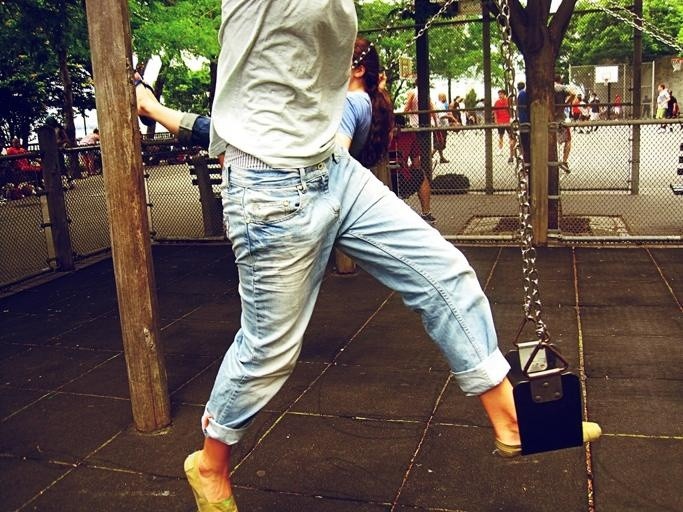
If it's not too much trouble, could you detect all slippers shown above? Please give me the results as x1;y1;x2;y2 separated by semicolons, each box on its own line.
134;68;158;126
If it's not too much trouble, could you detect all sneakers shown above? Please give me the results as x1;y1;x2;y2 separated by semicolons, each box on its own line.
561;162;570;173
439;157;450;164
494;421;602;458
184;450;237;511
421;212;437;224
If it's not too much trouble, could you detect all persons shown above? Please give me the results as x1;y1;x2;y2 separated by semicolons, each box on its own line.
1;116;101;199
132;36;393;171
181;0;602;511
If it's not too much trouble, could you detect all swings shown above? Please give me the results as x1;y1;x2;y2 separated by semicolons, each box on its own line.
496;0;583;455
